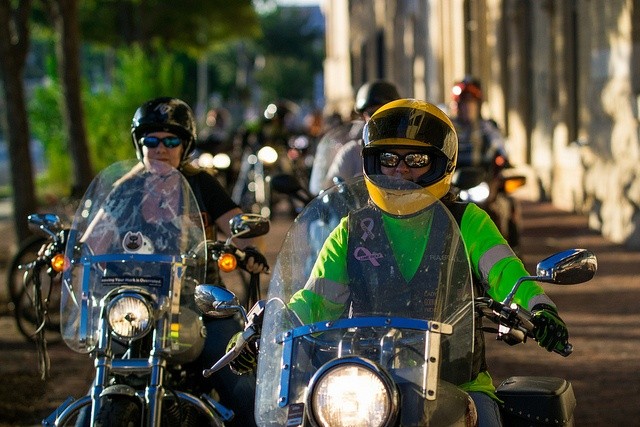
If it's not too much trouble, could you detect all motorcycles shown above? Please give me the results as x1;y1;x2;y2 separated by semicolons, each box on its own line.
263;169;367;282
193;171;599;427
16;157;273;427
447;153;527;250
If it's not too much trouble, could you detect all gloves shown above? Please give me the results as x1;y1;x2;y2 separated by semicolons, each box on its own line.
226;327;262;374
531;303;569;352
243;246;270;275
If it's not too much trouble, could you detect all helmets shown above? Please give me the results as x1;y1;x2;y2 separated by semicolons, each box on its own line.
355;80;400;116
362;98;459;218
131;97;198;157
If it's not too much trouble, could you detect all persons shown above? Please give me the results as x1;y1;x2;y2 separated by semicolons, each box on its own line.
40;96;271;427
224;98;570;426
441;76;519;249
309;81;404;196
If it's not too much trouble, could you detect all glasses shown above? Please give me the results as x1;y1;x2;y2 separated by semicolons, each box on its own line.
138;136;183;148
378;150;432;168
366;110;375;116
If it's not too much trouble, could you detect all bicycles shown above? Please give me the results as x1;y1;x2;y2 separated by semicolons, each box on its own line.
8;237;81;332
